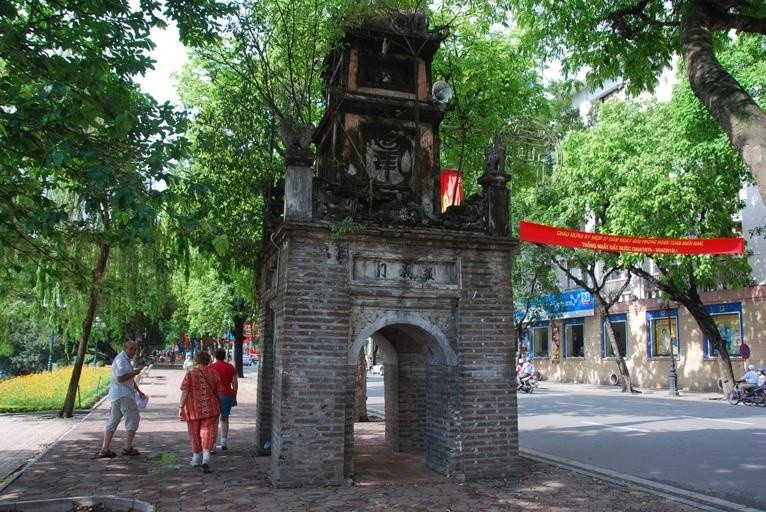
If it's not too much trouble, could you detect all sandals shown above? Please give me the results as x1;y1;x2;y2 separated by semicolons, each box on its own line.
98;448;118;458
120;446;140;456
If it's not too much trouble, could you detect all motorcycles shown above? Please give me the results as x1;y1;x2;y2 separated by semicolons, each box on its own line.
726;380;766;407
516;375;537;395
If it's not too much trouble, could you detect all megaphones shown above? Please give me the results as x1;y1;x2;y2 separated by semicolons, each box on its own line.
432;81;453;103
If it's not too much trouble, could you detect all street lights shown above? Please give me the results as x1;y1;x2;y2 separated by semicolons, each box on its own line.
655;297;682;398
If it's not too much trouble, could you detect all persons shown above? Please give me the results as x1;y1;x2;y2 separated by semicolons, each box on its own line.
98;340;145;458
516;358;534;390
739;364;766;400
178;349;237;473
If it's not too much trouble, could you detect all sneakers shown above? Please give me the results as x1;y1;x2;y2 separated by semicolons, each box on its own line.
191;457;212;474
